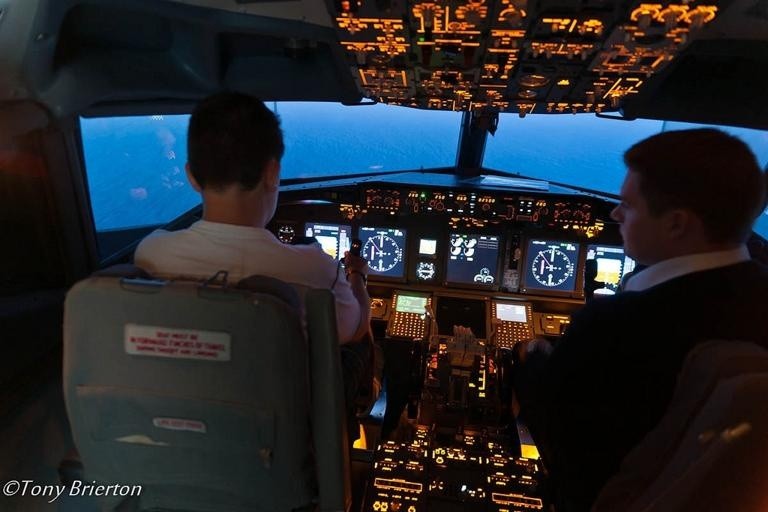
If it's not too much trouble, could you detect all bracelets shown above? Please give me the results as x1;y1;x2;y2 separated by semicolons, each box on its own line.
346;267;369;287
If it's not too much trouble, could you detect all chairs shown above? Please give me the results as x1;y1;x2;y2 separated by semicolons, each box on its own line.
587;340;767;509
58;275;357;511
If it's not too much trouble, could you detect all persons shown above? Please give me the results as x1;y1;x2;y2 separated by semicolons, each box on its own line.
511;124;767;510
134;88;387;417
